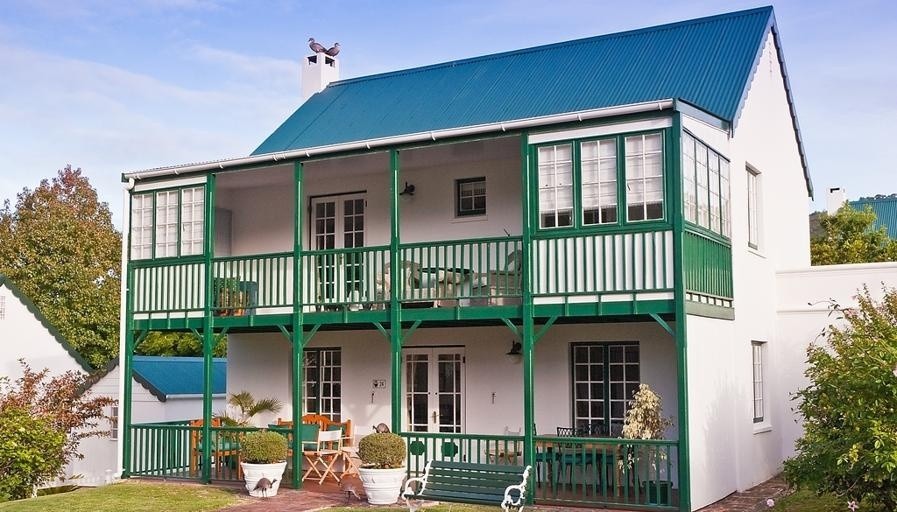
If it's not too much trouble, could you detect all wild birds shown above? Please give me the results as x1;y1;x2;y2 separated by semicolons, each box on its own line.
325;40;341;57
252;478;278;499
308;36;326;54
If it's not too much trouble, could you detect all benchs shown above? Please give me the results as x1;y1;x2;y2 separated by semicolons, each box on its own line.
188;417;244;479
400;458;532;511
275;414;351;478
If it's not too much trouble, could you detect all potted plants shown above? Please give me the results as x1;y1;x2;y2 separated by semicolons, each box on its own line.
237;430;288;497
355;432;407;504
616;383;674;505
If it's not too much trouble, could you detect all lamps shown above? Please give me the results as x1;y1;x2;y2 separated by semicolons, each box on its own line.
504;339;523;363
399;179;416;203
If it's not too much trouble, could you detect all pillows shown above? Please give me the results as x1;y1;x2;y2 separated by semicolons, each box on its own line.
267;423;319;450
194;428;241;451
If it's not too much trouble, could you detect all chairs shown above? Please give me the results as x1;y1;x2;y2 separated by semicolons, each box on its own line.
301;423;374;484
383;250;523;308
484;422;641;497
